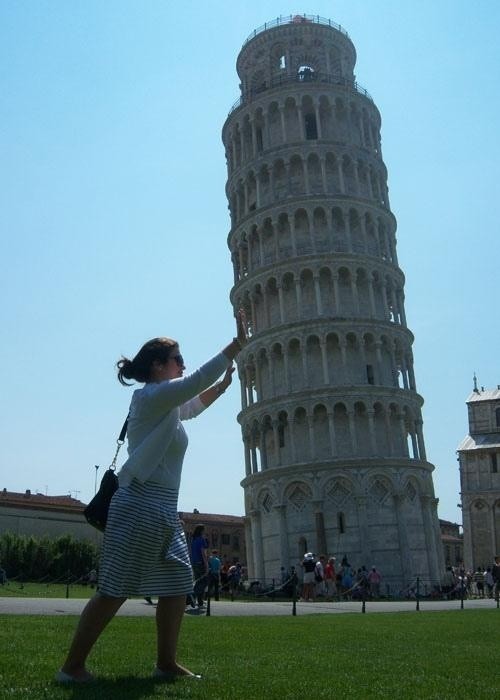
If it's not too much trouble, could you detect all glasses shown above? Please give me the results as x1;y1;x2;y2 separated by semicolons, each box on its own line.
168;354;184;367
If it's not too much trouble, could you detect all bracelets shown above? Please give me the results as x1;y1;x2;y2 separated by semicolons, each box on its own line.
233;337;244;351
214;383;226;394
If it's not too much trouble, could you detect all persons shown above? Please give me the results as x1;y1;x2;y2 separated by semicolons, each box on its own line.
54;307;249;684
203;548;500;602
144;595;153;605
187;523;209;609
87;568;97;588
79;567;90;586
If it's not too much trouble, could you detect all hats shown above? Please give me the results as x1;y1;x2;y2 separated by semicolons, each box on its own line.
304;552;314;561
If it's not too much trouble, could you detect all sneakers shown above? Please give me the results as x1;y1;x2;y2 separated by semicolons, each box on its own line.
188;594;196;608
150;662;202;680
199;605;207;609
55;666;97;688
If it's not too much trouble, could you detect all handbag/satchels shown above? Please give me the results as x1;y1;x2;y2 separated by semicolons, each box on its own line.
314;574;323;583
83;469;119;532
